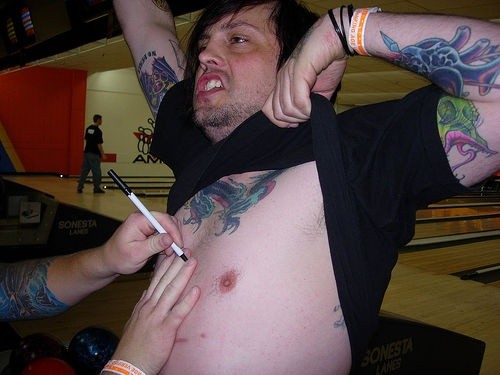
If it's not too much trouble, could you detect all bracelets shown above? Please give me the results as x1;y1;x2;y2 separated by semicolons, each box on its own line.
327;4;382;57
99;359;146;375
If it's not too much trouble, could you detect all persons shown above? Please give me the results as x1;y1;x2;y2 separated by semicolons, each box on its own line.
0;211;202;375
113;0;500;375
77;114;106;194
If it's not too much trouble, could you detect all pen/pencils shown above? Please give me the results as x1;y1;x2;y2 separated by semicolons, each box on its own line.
108;169;189;264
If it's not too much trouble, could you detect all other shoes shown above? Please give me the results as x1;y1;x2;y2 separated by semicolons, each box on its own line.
78;186;82;193
94;189;105;193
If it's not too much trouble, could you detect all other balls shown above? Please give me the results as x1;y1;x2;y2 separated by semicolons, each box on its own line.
22;357;76;375
68;325;120;375
9;332;68;375
